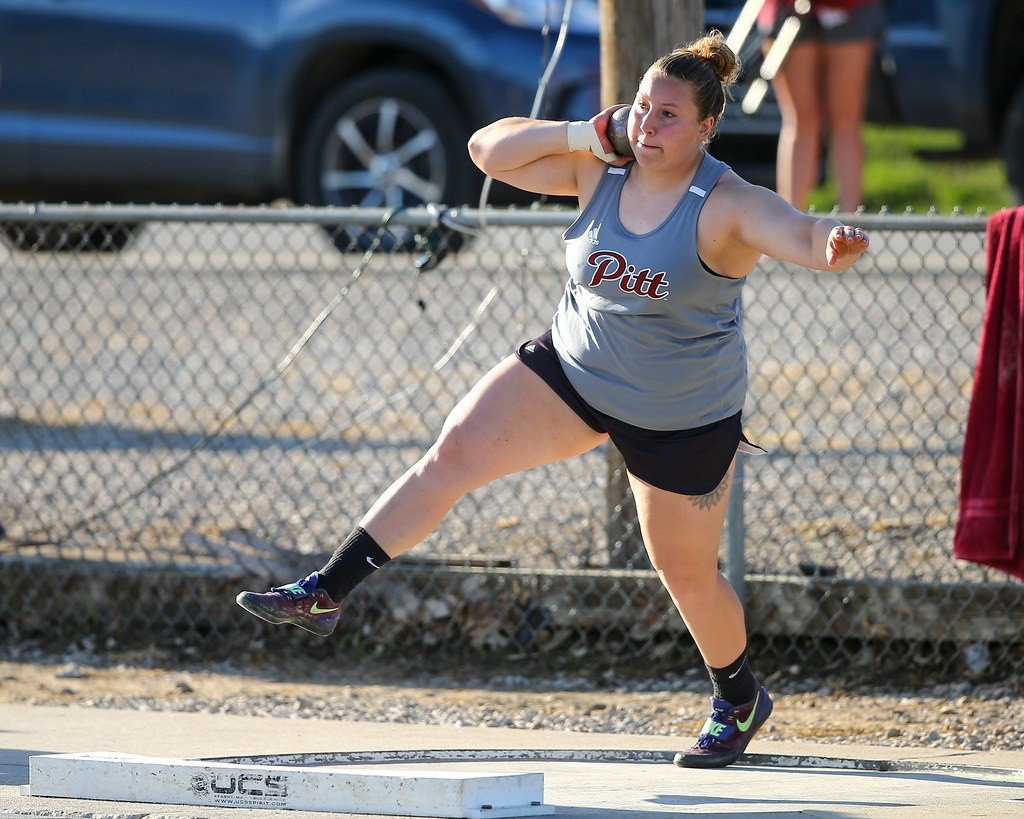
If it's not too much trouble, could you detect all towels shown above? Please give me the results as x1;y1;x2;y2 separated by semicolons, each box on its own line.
952;205;1024;582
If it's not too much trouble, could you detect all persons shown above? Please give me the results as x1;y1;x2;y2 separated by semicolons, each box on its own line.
756;0;882;212
237;30;871;772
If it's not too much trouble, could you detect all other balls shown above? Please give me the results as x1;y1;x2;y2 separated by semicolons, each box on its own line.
606;105;635;157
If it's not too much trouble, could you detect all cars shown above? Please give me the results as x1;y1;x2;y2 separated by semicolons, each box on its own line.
702;0;991;190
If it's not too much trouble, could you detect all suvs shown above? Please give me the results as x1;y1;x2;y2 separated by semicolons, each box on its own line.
0;0;600;254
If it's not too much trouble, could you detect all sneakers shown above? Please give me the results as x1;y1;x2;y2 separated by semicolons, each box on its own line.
672;679;773;768
236;571;343;638
